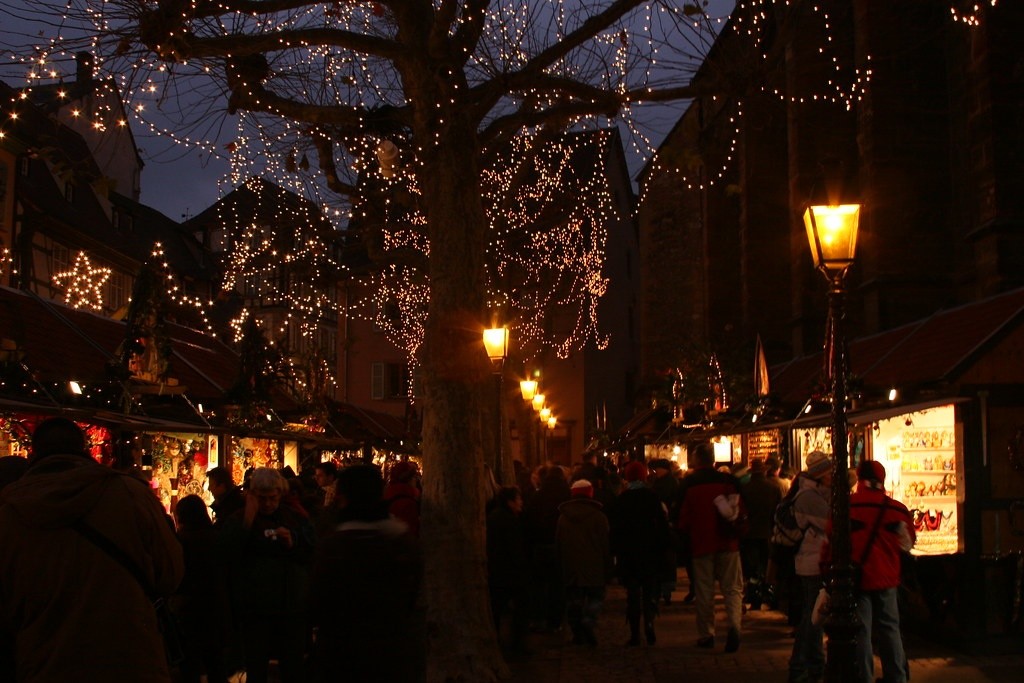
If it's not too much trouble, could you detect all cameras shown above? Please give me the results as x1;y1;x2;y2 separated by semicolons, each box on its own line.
264;529;278;541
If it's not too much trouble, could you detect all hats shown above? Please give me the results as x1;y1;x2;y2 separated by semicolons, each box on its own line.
856;457;886;489
807;452;835;479
746;454;783;478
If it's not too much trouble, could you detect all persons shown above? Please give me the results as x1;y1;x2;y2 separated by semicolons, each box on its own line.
0;418;915;683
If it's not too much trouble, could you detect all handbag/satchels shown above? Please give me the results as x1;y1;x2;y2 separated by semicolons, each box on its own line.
819;558;871;599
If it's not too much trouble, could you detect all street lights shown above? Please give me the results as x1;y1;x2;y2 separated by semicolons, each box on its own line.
520;379;558;468
483;327;511;491
803;193;868;683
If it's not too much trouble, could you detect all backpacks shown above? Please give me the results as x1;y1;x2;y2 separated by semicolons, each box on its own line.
768;484;833;571
712;491;756;542
